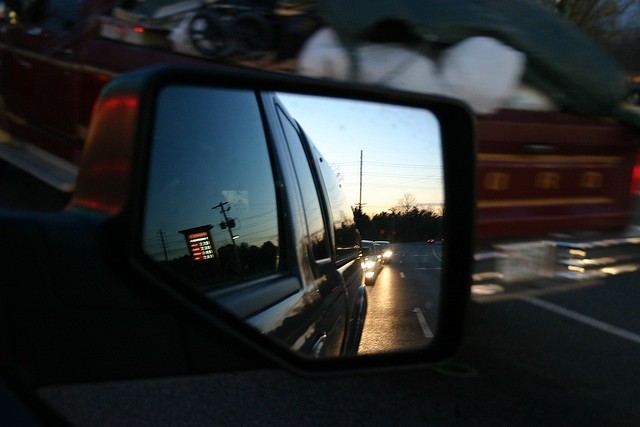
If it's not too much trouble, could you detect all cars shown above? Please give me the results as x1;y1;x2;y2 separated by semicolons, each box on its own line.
0;0;639;306
373;242;383;267
143;87;367;354
357;239;377;284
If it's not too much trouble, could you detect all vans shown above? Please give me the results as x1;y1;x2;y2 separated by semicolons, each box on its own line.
375;239;392;263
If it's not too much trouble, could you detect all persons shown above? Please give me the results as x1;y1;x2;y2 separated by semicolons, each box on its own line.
227;15;323;73
